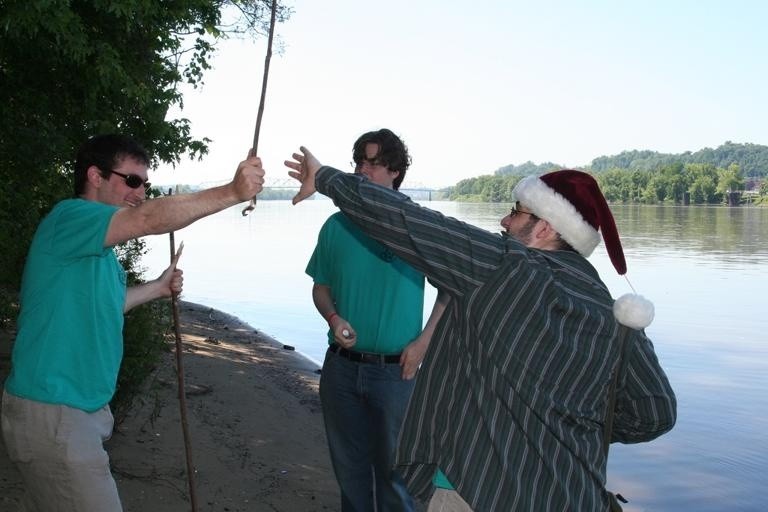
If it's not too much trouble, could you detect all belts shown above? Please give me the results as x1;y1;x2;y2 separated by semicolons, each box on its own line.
328;342;401;365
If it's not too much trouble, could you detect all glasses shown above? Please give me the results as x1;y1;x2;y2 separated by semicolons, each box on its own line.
100;166;152;194
349;159;388;171
510;207;540;220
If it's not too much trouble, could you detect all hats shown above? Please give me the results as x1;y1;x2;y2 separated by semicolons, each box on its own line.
509;168;657;333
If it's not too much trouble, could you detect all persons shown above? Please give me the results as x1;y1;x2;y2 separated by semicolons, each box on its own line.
0;134;266;512
284;146;677;512
305;130;452;512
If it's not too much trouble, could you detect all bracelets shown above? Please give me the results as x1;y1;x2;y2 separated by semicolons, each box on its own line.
327;313;338;326
